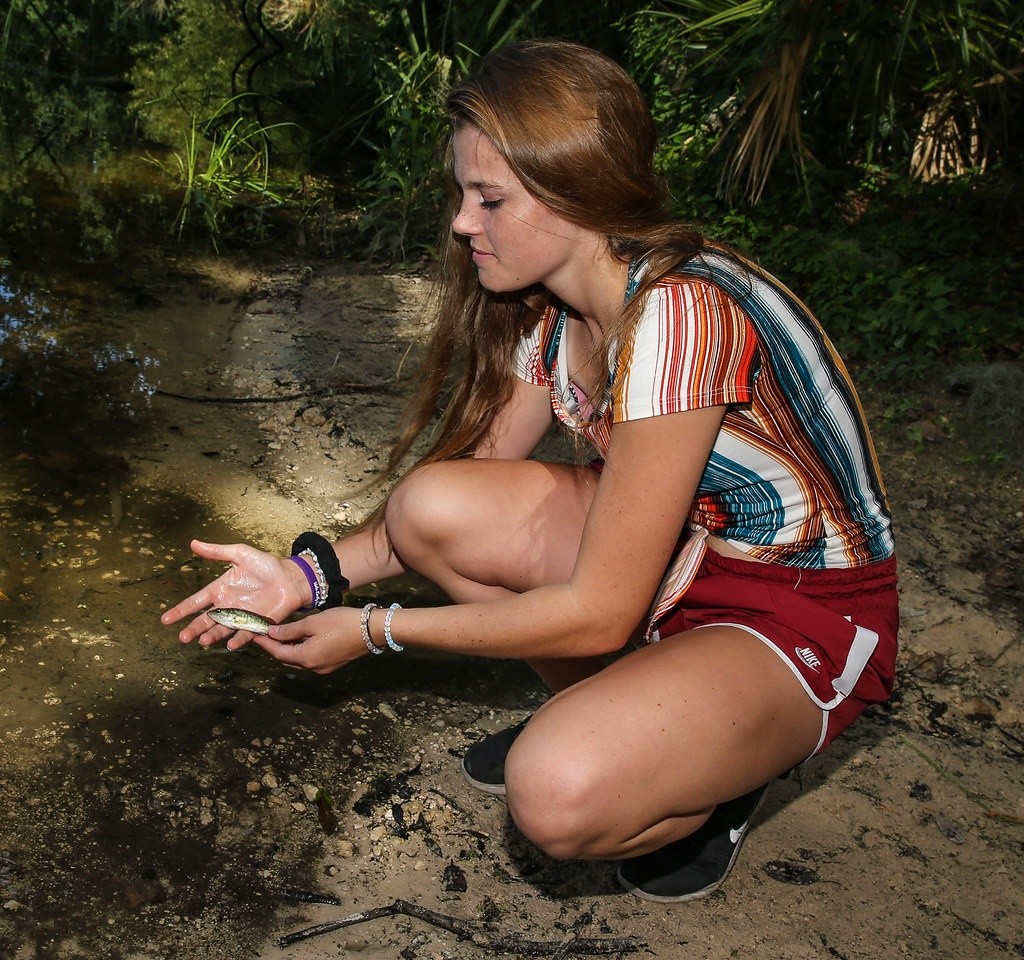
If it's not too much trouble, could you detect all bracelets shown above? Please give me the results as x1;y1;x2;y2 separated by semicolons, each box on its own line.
298;548;329;606
384;603;404;652
360;603;385;654
287;556;321;614
291;531;350;610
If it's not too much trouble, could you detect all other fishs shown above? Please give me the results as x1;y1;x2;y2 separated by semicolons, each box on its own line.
208;608;278;635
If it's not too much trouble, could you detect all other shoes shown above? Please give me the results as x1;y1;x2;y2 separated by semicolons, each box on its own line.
464;705;539;791
616;779;772;904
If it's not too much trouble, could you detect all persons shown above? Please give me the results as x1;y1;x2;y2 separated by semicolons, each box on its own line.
160;35;901;904
